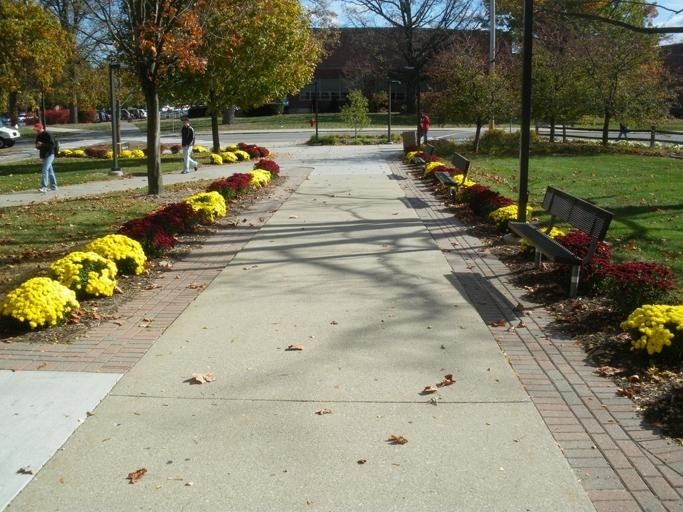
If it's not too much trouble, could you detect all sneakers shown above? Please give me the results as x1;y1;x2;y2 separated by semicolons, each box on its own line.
36;185;57;193
180;161;198;174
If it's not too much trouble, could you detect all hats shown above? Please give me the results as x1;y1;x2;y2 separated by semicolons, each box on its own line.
31;122;44;132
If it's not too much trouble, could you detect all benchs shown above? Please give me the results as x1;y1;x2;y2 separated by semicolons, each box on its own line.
507;186;615;297
434;152;469;203
412;143;433;165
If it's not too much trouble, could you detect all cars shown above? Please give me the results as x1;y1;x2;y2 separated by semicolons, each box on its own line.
0;111;34;149
96;105;190;123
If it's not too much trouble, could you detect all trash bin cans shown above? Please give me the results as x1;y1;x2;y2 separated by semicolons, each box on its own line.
400;131;418;152
222;109;235;124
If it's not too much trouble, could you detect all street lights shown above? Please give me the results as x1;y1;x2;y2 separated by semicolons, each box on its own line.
388;78;401;142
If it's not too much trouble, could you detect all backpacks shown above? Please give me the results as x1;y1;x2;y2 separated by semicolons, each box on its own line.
52;135;60;157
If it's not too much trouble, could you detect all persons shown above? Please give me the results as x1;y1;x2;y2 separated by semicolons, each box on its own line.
615;123;628;142
180;116;198;174
420;111;431;144
32;122;57;193
